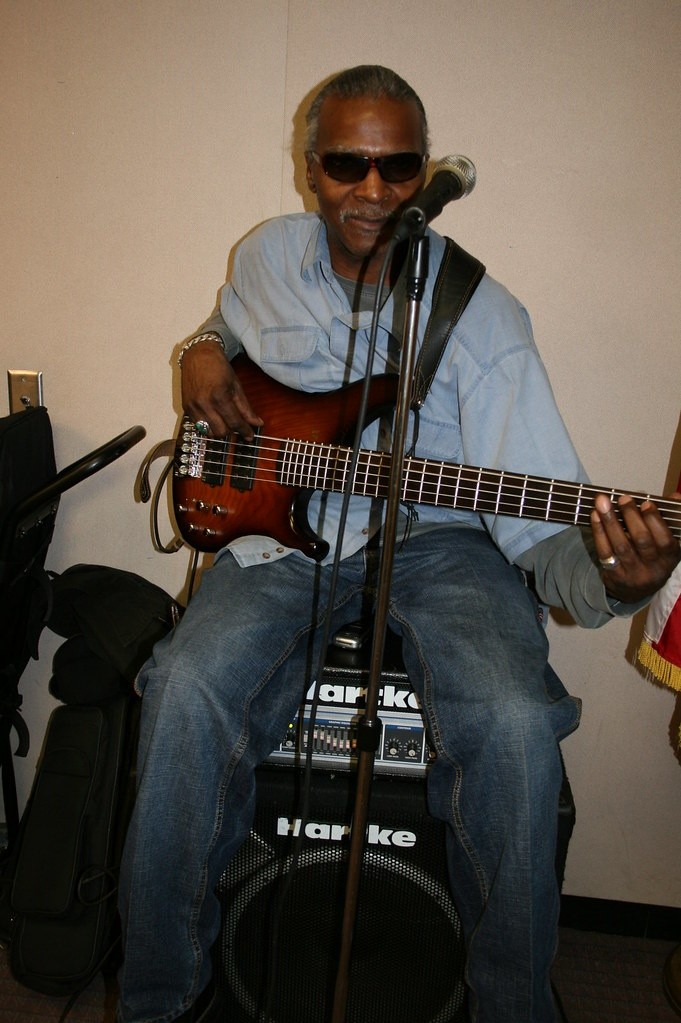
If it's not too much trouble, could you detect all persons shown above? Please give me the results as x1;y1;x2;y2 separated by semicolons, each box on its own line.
112;66;681;1023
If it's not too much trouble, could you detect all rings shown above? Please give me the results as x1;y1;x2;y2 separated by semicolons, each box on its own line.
196;420;209;433
600;554;618;569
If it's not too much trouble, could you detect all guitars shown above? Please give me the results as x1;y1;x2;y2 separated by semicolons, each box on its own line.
168;345;680;565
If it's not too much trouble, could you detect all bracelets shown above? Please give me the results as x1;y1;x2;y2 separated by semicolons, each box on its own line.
177;334;224;368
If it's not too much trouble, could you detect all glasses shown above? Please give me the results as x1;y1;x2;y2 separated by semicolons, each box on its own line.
312;150;426;184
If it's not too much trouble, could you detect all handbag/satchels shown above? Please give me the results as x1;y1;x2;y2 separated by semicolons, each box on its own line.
44;562;184;678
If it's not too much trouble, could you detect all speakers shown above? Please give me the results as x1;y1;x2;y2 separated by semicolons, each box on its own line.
195;760;577;1023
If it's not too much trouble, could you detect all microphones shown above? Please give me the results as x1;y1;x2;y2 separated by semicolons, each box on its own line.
393;155;477;244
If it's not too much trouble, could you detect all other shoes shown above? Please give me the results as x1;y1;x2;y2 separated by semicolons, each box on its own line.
172;978;223;1023
